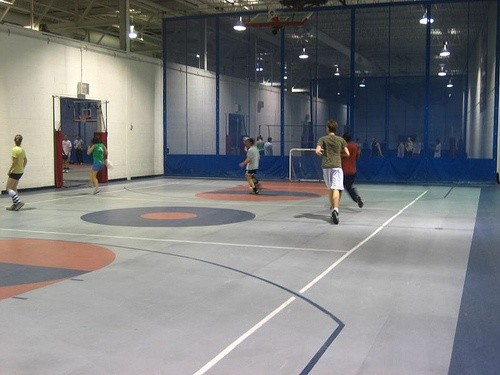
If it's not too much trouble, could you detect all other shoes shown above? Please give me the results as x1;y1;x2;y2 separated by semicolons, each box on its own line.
356;196;363;207
63;169;65;173
66;170;69;173
94;188;101;194
6;204;17;210
15;201;24;211
332;210;339;224
254;189;259;194
254;182;259;190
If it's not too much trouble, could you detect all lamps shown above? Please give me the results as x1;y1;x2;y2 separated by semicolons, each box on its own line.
299;48;308;59
419;9;433;24
234;16;246;31
440;42;450;57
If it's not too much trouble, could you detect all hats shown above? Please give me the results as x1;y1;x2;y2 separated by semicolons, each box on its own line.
76;136;80;139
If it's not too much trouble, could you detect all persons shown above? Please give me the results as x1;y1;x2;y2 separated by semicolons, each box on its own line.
355;136;443;160
87;136;107;194
73;135;85;164
5;134;27;211
239;137;260;194
264;137;273;156
315;120;350;224
256;136;265;155
61;134;72;173
339;133;364;208
